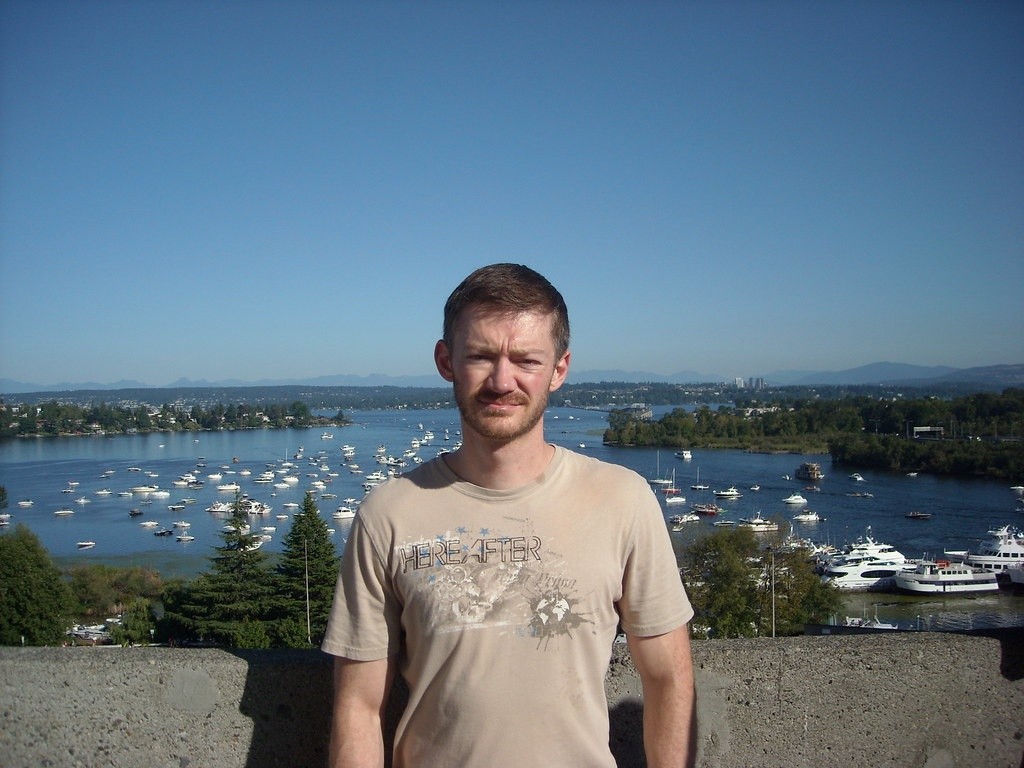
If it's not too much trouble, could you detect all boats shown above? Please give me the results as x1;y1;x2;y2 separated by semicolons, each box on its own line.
68;481;79;487
1009;485;1024;514
0;514;12;526
906;472;918;477
895;552;1001;593
99;474;109;478
92;488;114;496
646;446;908;596
53;510;75;516
17;499;34;506
904;511;931;520
942;525;1024;587
104;467;116;474
74;498;91;504
119;425;464;554
77;546;93;551
61;488;74;493
76;542;95;546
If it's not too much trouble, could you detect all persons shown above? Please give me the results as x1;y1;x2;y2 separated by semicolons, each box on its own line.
320;263;696;768
